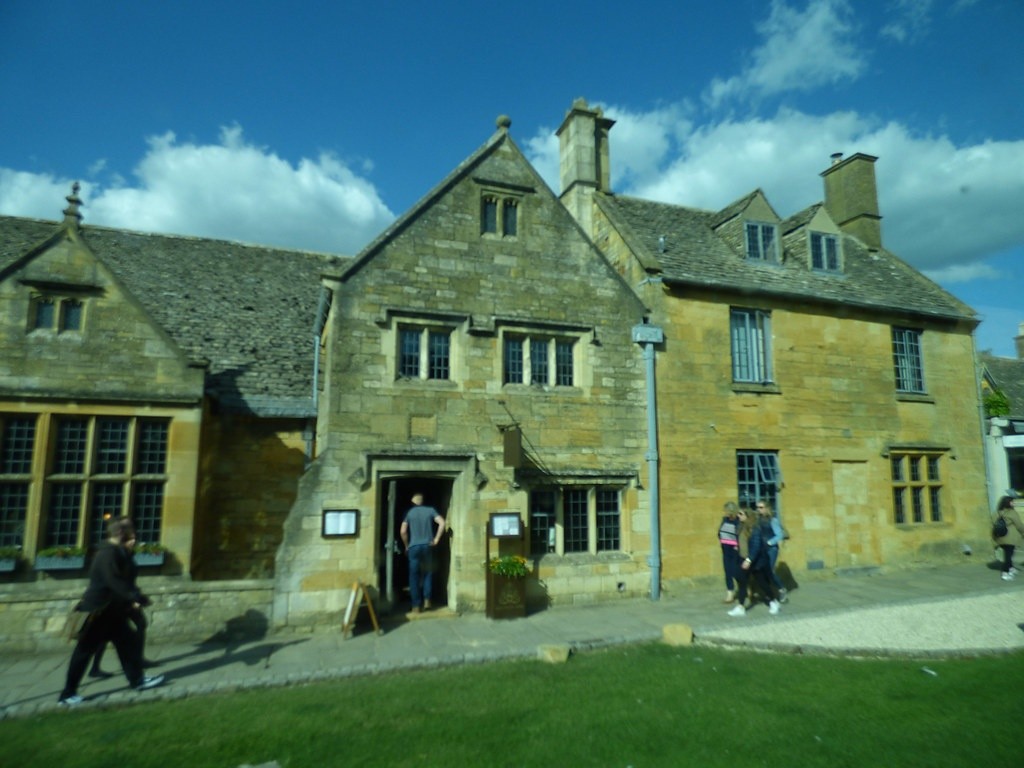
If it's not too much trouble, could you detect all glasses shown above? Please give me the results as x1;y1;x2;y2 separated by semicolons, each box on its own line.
739;513;746;515
756;506;764;509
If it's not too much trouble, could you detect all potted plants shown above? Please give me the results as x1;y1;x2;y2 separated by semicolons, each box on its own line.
483;554;534;595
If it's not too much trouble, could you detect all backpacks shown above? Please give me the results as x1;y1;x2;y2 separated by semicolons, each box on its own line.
993;510;1013;536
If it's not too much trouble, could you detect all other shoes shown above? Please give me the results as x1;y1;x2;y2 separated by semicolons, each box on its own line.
142;660;156;667
89;669;113;678
58;696;82;706
411;606;421;613
424;600;431;608
1009;567;1019;574
743;597;751;608
136;675;164;690
728;606;745;616
768;600;780;614
1001;571;1012;580
726;590;737;603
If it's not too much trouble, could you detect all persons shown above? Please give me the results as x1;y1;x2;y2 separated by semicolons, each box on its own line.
755;500;787;594
728;507;779;617
401;493;445;614
55;516;165;707
717;502;756;603
990;497;1024;581
